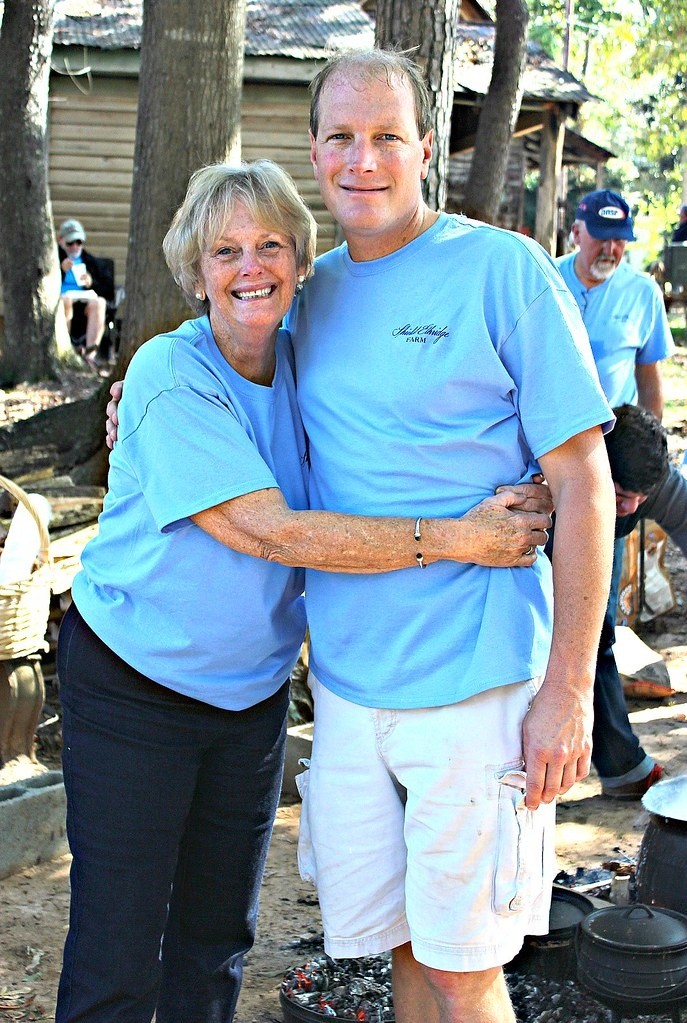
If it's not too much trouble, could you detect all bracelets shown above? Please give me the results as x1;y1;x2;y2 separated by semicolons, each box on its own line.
413;517;427;569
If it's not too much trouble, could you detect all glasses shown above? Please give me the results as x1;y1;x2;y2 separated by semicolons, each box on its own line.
66;240;82;245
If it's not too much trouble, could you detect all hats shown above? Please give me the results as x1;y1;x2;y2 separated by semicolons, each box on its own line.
576;187;635;241
60;219;85;244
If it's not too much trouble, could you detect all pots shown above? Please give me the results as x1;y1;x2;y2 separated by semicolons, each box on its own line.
631;774;686;917
576;905;687;1014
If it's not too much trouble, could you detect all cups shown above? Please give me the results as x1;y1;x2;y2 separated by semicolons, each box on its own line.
71;263;87;286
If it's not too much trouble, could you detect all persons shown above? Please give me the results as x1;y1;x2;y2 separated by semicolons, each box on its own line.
557;189;687;796
26;159;553;1022
57;219;106;369
104;48;616;1023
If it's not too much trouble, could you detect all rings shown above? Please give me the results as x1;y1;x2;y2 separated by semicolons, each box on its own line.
525;545;534;555
539;528;549;546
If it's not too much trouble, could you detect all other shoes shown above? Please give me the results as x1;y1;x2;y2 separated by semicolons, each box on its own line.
603;762;672;798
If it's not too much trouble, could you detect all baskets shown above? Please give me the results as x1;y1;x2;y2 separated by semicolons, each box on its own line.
0;474;53;661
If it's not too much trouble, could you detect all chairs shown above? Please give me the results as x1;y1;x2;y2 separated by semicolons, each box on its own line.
70;257;118;362
